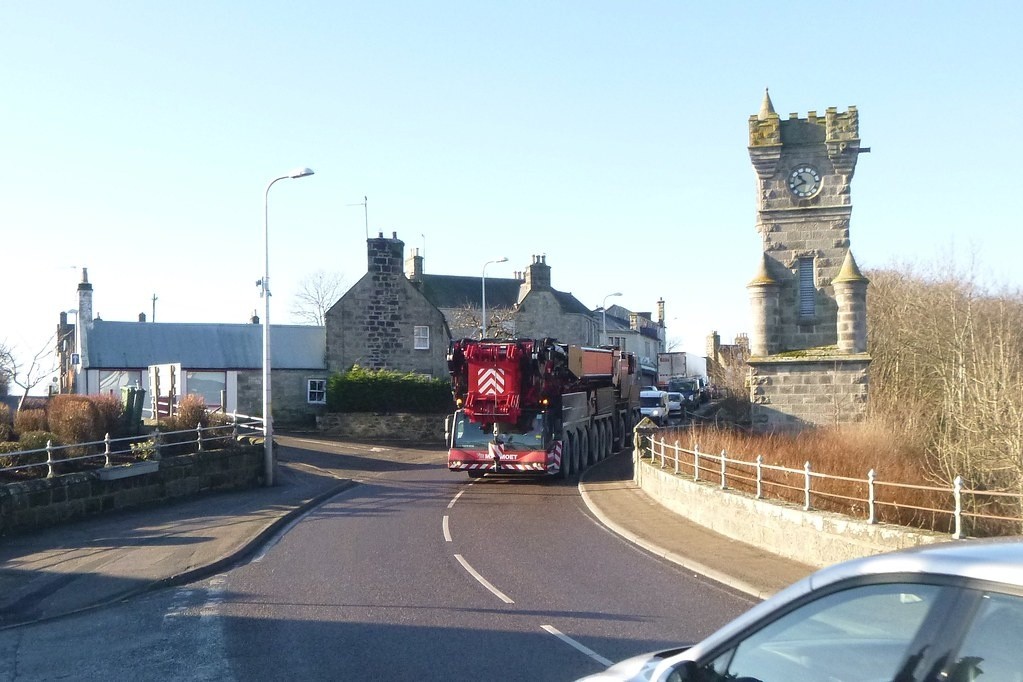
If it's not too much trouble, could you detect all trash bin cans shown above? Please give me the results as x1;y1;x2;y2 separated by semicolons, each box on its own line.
120;385;146;425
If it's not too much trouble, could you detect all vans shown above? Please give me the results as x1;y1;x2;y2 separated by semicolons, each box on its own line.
638;390;671;427
641;386;658;392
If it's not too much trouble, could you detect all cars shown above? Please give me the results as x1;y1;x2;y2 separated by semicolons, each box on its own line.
667;391;686;412
567;531;1023;682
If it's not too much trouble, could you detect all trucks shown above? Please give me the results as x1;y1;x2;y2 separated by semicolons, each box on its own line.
669;377;712;412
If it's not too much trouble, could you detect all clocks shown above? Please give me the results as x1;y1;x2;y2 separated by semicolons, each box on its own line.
788;166;820;197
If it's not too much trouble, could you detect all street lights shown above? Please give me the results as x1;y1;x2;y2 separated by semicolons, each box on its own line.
481;258;508;338
603;293;623;344
261;168;315;487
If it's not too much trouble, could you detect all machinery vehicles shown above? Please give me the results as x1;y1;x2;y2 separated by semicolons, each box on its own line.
443;335;641;480
